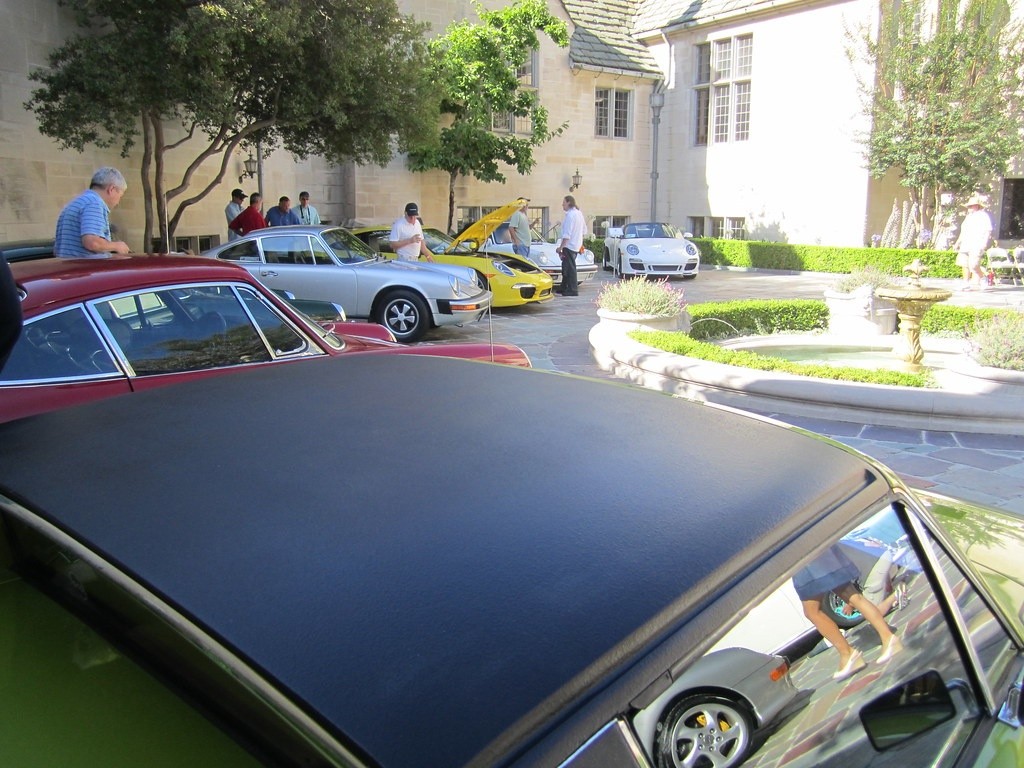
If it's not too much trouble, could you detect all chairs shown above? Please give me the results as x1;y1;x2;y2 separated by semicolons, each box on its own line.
267;247;279;263
293;243;305;264
985;246;1024;286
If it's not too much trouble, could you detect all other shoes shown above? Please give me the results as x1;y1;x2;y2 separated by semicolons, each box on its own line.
562;291;577;296
556;288;565;293
894;581;908;609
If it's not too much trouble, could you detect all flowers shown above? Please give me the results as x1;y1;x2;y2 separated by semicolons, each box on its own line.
591;271;688;317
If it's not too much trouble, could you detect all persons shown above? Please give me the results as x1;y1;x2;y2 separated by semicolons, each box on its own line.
292;191;321;225
264;196;304;226
54;167;131;257
556;195;587;296
389;203;435;263
954;196;992;291
225;189;248;242
230;192;268;237
0;252;24;379
509;196;541;256
792;540;908;680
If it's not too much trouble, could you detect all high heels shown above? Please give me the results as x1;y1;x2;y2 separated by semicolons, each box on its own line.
833;649;865;683
876;634;904;664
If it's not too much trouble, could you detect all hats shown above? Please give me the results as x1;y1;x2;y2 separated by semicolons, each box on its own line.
518;197;530;201
405;203;418;215
232;189;248;199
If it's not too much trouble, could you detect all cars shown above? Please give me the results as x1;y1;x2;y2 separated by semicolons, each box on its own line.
0;240;346;348
697;527;913;658
602;222;700;279
198;225;494;344
305;197;554;307
0;353;1023;768
632;647;797;768
477;222;598;288
1;258;533;424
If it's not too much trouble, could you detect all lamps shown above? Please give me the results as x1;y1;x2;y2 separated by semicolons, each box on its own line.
569;168;582;192
238;150;256;183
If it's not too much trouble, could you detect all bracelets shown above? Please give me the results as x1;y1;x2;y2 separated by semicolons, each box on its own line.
426;255;430;258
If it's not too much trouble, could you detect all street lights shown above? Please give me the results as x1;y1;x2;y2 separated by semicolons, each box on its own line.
650;93;664;222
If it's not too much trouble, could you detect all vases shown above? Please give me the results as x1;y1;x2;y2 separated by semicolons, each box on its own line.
595;307;677;331
